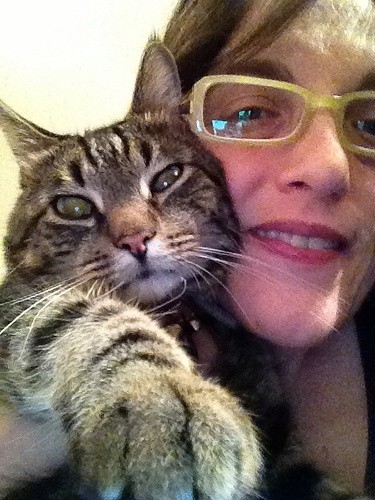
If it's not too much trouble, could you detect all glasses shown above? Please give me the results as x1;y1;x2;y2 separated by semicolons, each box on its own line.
179;75;375;158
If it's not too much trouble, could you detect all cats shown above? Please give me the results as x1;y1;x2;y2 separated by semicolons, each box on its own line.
0;27;354;500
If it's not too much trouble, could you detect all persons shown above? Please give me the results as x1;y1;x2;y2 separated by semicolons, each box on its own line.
0;0;375;500
